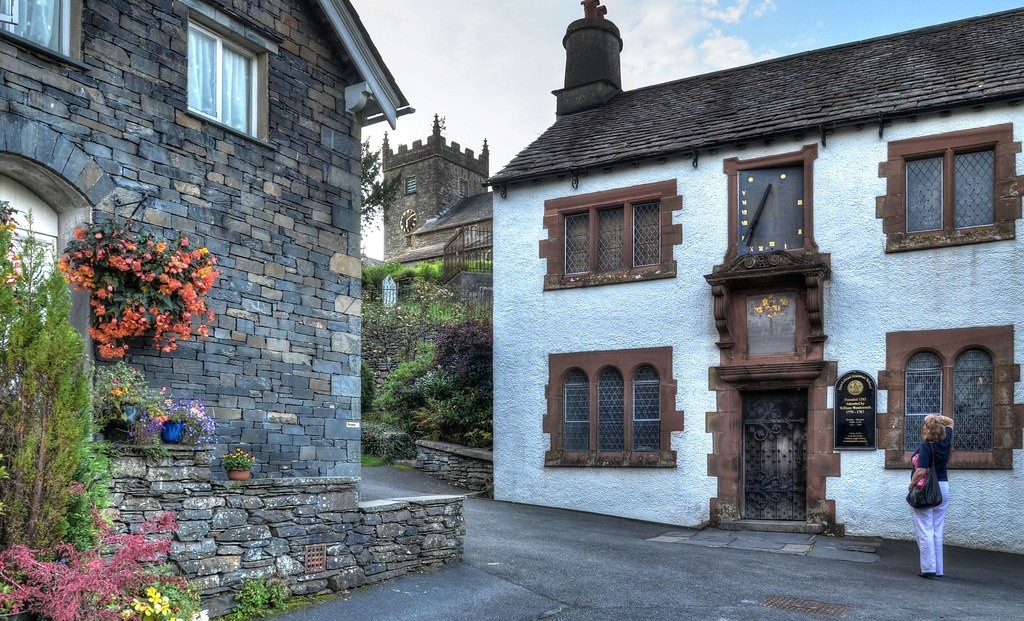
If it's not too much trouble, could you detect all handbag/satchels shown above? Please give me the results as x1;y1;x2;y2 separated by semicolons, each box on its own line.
905;442;943;509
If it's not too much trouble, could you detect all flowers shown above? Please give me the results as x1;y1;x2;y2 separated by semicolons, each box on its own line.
117;391;222;465
220;447;256;470
86;360;165;431
58;217;221;360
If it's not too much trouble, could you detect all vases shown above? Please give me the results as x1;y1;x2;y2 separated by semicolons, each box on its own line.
228;469;250;480
161;421;185;444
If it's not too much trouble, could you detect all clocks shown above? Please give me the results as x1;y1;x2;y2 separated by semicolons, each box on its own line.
400;209;417;232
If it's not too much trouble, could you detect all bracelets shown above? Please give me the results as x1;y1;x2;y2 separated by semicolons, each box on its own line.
941;416;944;423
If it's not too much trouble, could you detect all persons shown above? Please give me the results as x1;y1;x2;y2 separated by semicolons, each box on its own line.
905;414;955;580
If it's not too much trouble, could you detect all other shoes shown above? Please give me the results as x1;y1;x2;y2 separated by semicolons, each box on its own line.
918;572;936;578
